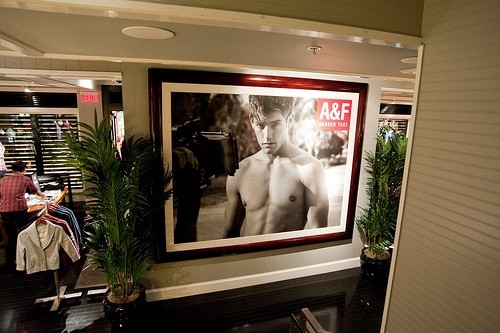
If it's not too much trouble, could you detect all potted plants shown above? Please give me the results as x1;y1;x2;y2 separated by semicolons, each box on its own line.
356;124;409;283
54;107;174;333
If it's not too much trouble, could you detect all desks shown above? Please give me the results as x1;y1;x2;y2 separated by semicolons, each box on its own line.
24;188;68;212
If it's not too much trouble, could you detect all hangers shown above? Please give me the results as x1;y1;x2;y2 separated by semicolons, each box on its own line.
33;202;59;226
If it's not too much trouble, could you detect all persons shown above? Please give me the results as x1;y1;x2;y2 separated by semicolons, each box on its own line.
0;142;46;261
222;95;330;238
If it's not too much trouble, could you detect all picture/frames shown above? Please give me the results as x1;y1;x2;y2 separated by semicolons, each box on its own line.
148;67;369;264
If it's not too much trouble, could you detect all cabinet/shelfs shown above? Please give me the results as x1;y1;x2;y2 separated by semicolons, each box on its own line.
378;119;408;139
